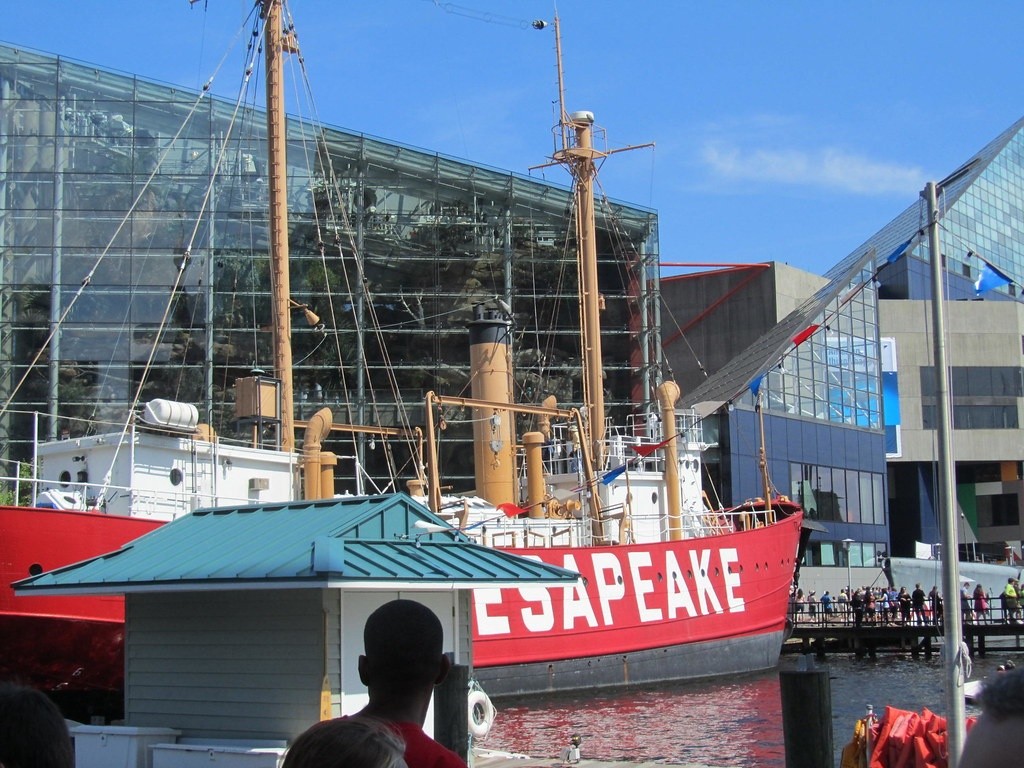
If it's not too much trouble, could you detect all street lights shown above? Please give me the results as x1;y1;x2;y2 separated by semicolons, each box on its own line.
843;538;856;601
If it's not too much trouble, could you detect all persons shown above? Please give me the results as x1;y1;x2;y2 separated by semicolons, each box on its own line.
280;599;469;767
795;577;1023;626
1;668;76;768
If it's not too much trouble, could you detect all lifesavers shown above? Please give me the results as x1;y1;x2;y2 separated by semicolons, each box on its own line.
466;690;495;737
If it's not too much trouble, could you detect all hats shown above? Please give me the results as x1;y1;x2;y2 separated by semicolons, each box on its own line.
810;591;816;596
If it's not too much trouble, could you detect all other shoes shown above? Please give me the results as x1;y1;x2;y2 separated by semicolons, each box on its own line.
809;618;815;622
814;618;818;622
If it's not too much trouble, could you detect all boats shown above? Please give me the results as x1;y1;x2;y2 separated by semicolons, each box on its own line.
1;0;804;700
